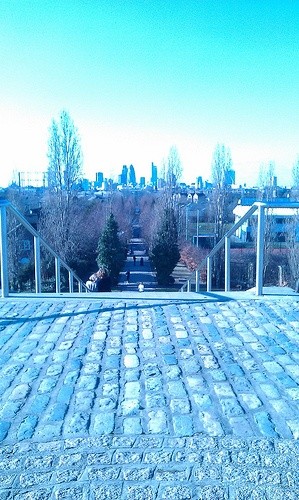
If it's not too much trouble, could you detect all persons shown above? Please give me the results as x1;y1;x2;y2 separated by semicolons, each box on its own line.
137;282;145;292
133;256;137;265
125;270;130;281
140;257;144;265
